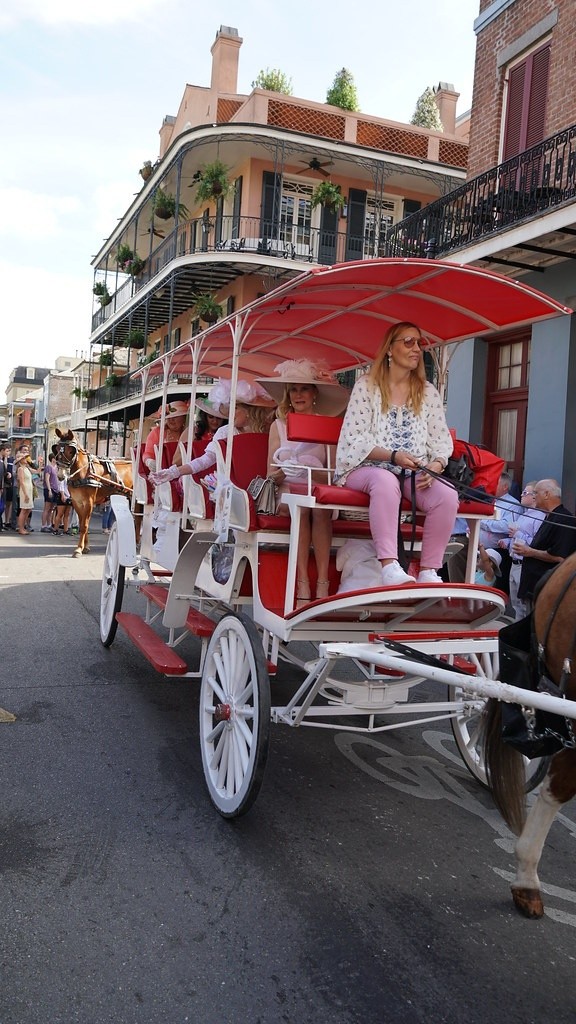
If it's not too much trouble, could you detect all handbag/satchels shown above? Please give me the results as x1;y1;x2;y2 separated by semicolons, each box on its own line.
246;475;277;516
442;457;473;493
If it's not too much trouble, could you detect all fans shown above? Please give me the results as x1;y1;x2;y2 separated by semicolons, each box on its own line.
295;157;336;178
138;227;165;239
180;170;203;188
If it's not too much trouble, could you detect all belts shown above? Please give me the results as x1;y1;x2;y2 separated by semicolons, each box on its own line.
512;560;523;564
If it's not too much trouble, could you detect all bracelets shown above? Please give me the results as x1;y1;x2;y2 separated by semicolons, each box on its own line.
391;450;398;466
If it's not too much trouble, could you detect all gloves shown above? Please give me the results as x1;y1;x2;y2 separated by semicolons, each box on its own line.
147;464;179;485
145;459;158;471
281;460;301;478
289;466;306;479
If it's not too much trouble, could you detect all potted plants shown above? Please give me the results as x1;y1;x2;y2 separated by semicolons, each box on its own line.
96;295;113;306
123;329;153;351
116;243;143;274
191;293;224;325
308;181;344;211
195;160;238;207
136;160;154;178
104;373;122;387
92;282;109;295
152;189;192;223
98;354;117;366
72;386;95;398
136;351;158;367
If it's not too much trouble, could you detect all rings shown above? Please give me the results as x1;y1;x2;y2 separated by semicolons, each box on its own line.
428;483;431;487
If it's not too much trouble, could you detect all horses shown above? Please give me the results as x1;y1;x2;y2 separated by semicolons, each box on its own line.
54;428;144;558
483;552;576;920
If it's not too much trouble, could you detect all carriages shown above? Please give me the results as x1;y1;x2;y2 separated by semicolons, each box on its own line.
98;256;576;920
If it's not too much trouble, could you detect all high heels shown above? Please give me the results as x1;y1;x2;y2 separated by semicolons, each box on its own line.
296;580;311;609
315;580;329;600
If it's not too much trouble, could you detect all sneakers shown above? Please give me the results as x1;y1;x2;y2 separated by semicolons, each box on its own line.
417;569;444;584
381;561;416;586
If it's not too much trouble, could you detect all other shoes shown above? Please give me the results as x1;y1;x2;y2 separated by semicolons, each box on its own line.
0;519;114;535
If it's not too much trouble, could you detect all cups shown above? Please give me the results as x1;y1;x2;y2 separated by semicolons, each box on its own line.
514;538;526;545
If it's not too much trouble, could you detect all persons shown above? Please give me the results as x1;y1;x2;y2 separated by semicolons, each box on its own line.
42;453;80;536
148;377;278;502
171;397;229;493
438;472;523;596
253;358;351;609
512;479;576;601
497;481;547;620
0;443;44;535
142;401;189;473
102;507;115;534
333;320;459;585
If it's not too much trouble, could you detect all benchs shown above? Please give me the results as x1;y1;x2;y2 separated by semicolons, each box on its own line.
153;441;188;514
279;409;505;525
214;430;429;550
129;446;157;515
179;440;226;534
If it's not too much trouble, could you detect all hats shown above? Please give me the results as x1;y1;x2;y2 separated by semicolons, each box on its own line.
12;452;30;465
152;405;173;422
477;548;502;577
165;400;190;419
194;392;229;420
253;357;349;417
208;377;278;408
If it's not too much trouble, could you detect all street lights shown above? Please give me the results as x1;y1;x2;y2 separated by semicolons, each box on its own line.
42;417;49;479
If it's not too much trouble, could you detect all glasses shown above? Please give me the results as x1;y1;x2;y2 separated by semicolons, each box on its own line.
522;491;535;496
391;337;430;351
